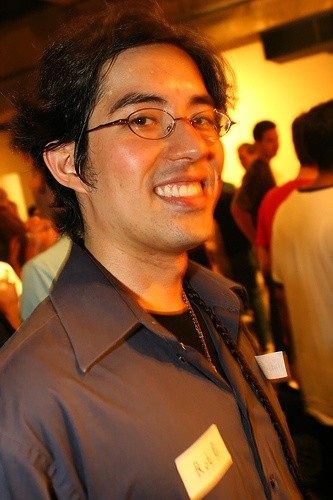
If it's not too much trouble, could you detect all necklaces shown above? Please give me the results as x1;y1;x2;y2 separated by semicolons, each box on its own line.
181;290;218;374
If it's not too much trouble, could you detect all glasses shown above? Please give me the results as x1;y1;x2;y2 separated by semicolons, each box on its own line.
88;107;236;141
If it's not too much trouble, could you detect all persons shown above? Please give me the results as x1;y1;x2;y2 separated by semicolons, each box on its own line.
189;99;333;500
0;184;73;348
0;10;312;500
